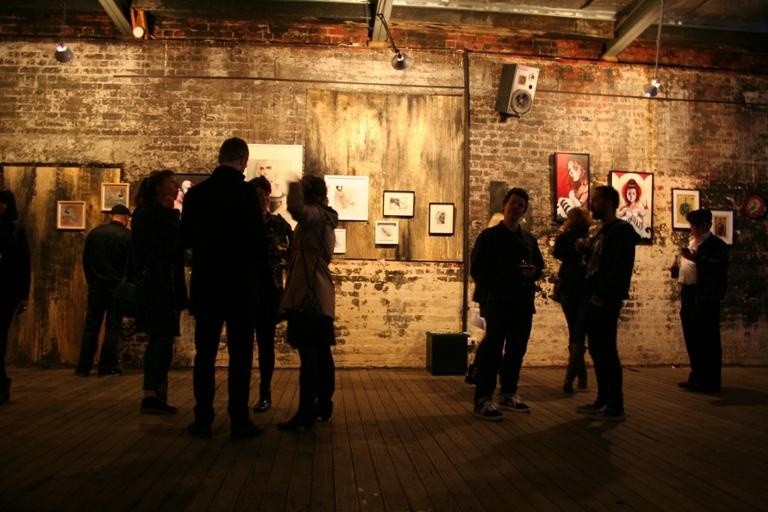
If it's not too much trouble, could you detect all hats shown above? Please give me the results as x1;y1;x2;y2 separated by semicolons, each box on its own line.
110;204;133;218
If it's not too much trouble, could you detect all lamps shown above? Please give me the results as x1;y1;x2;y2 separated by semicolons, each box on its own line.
376;13;412;70
644;1;664;98
57;2;73;63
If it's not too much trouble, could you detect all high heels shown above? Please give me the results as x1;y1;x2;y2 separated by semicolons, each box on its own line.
284;408;318;432
314;398;334;422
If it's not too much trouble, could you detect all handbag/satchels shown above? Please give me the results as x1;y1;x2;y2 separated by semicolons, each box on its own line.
111;276;149;318
286;302;328;350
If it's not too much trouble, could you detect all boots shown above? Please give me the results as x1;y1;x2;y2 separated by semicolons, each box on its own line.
252;382;274;414
0;377;14;405
563;343;587;393
577;362;587;389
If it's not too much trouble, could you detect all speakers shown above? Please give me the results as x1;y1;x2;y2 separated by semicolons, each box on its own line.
495;64;540;117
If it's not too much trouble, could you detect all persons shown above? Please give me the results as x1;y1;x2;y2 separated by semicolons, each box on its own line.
669;206;729;394
463;212;505;389
0;163;32;403
616;178;648;238
556;155;588;220
573;184;641;422
74;138;337;442
547;206;593;396
469;186;544;422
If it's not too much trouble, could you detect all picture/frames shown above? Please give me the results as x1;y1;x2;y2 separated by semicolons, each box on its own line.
332;228;346;255
243;143;304;232
554;152;590;222
171;174;211;221
709;207;734;245
382;190;415;218
608;170;654;245
56;201;86;231
323;175;369;221
375;221;399;245
671;188;700;231
429;203;455;235
101;182;130;212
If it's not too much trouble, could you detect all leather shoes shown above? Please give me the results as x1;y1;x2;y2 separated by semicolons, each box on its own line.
230;423;264;441
186;419;213;439
141;396;179;416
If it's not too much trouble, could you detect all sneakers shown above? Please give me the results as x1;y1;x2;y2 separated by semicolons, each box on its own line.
74;367;90;378
584;405;625;420
677;380;721;394
498;393;529;412
97;367;122;377
575;404;601;414
474;396;504;420
